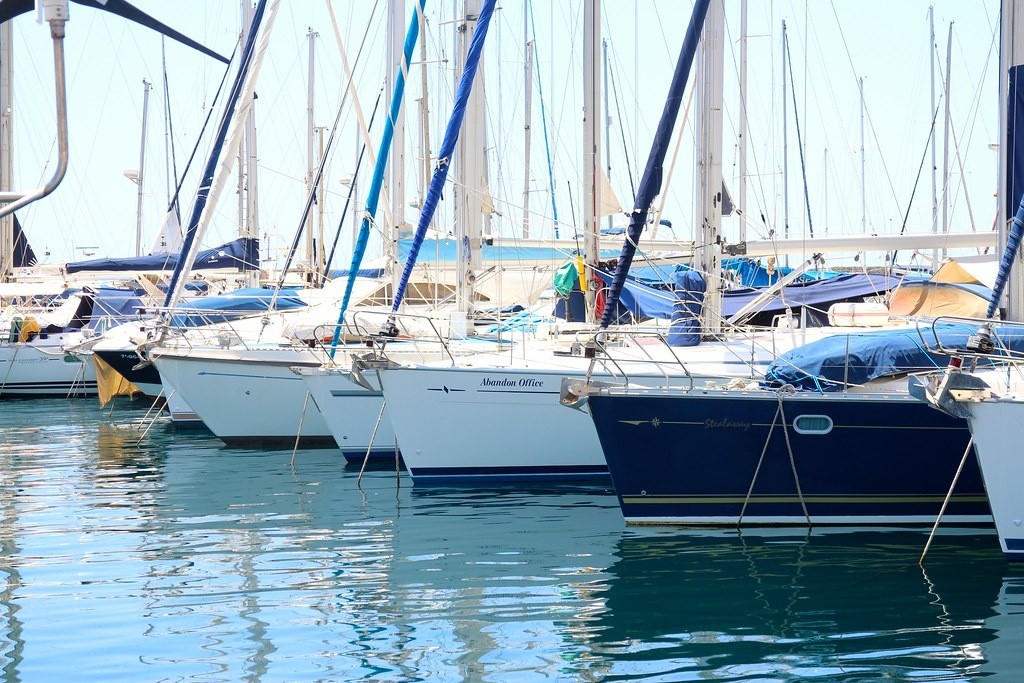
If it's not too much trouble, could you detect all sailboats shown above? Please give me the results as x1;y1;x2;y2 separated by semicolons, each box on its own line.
0;0;1024;557
